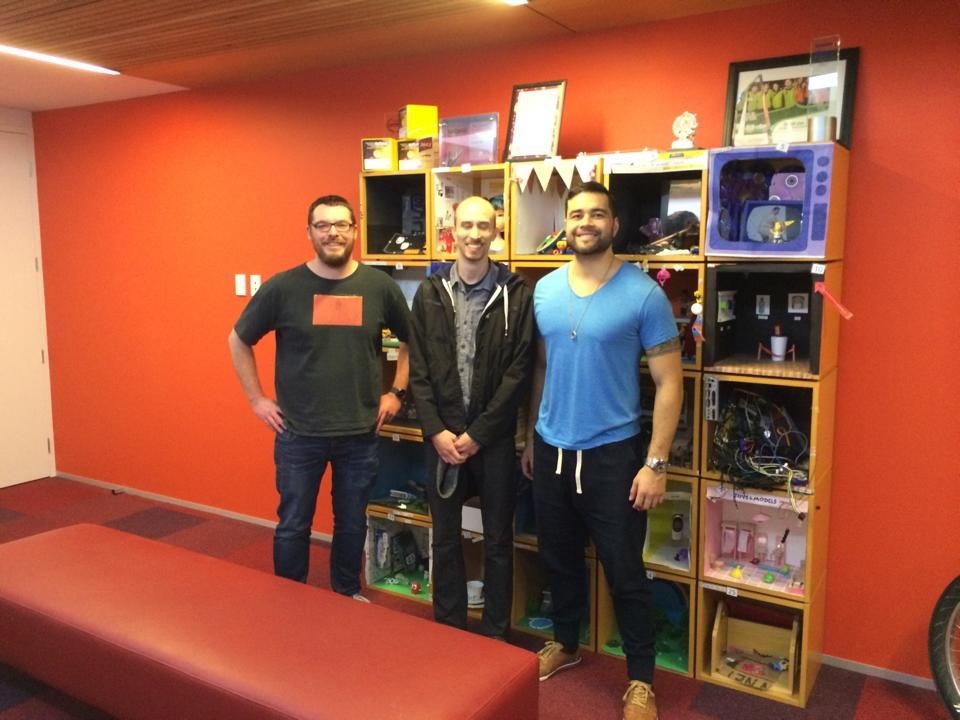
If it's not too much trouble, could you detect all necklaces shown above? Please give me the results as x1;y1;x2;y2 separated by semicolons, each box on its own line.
568;253;615;339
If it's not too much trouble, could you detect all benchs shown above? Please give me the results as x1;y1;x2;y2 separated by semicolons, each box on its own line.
1;521;539;720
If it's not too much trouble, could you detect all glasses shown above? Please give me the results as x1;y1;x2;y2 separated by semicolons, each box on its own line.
310;221;352;232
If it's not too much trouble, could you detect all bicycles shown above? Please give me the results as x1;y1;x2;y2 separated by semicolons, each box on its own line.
929;574;960;720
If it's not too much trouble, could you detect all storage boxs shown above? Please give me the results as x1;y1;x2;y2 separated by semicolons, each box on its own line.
361;137;399;171
398;139;440;170
399;104;438;139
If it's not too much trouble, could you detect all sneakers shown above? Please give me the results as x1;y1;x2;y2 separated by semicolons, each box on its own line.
622;680;659;720
535;639;582;681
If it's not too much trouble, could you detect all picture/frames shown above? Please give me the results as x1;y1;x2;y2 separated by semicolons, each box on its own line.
723;47;862;148
439;112;499;166
502;78;567;162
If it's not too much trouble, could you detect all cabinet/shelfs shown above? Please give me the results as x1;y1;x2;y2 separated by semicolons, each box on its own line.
358;141;852;709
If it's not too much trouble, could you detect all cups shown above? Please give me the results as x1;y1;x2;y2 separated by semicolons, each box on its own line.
466;581;484;603
771;336;788;361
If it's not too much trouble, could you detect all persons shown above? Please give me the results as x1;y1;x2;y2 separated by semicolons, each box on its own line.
228;195;409;603
521;181;682;720
410;196;536;642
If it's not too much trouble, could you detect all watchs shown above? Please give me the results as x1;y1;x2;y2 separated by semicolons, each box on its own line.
644;457;670;474
388;386;408;405
